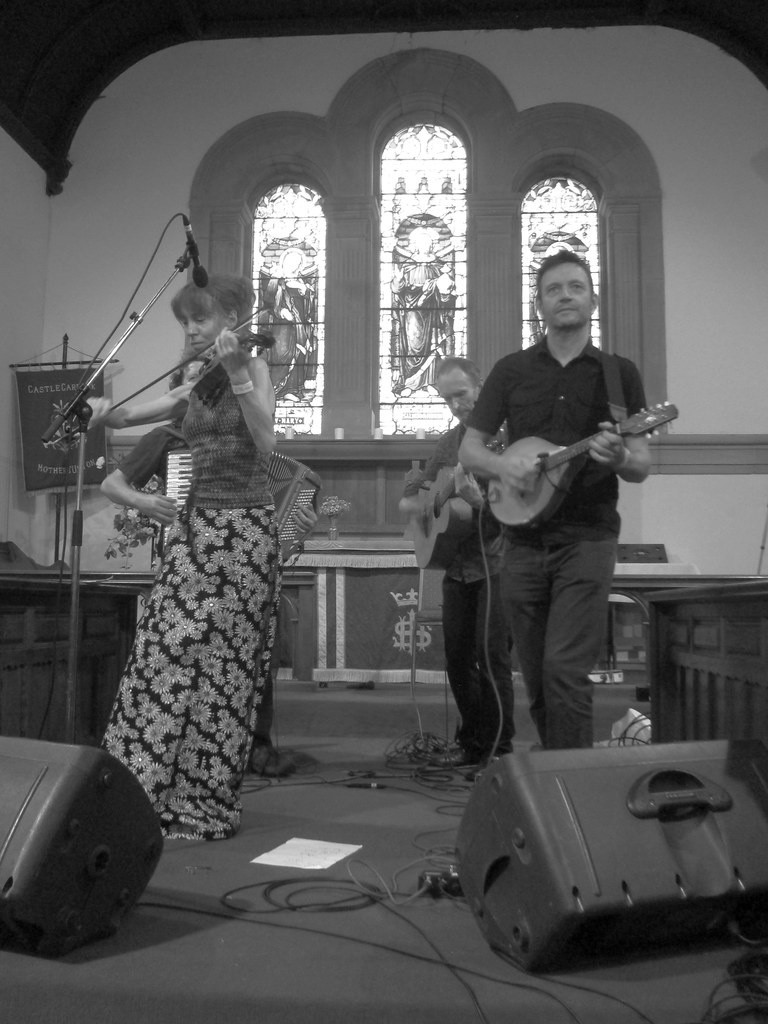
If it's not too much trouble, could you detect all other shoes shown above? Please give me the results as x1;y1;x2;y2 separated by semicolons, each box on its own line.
431;749;473;767
247;746;296;777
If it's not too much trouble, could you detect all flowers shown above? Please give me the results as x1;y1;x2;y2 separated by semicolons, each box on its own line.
318;494;350;516
94;455;165;571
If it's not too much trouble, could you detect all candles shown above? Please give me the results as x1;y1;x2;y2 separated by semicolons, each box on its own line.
374;428;383;440
334;426;345;440
285;428;296;441
415;429;425;440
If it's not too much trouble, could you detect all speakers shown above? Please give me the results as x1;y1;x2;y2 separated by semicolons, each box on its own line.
0;736;163;960
453;737;768;977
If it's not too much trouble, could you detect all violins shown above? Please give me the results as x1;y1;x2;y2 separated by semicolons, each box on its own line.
192;330;277;398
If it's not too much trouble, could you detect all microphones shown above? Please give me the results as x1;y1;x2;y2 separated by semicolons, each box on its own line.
181;215;209;288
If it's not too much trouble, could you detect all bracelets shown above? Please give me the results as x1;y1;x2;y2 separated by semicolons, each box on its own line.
613;446;631;473
232;380;254;394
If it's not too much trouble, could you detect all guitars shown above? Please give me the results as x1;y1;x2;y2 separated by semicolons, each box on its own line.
409;437;506;572
486;400;678;535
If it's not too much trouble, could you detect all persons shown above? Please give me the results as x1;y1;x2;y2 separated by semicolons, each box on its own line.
399;357;509;780
458;252;653;749
81;273;317;840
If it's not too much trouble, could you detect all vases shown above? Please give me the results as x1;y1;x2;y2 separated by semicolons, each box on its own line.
324;513;343;540
